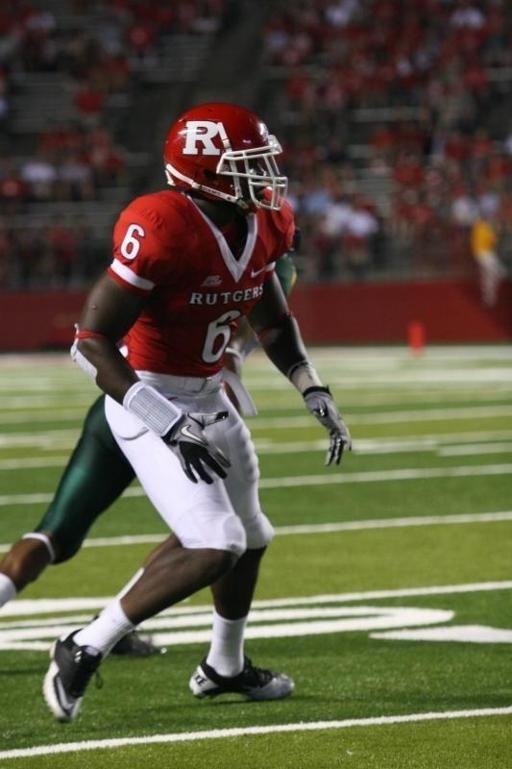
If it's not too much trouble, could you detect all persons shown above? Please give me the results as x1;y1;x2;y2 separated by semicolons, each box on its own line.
41;101;355;723
0;251;300;656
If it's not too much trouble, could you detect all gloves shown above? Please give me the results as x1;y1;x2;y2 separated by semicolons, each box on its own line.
160;411;233;484
302;387;352;467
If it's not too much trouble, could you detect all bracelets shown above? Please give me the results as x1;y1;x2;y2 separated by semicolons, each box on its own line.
121;380;182;438
286;359;324;395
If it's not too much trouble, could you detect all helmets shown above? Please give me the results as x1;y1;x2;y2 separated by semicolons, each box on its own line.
162;102;290;211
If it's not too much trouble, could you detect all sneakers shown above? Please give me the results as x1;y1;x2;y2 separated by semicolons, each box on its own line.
41;627;103;722
90;615;168;657
188;655;295;701
